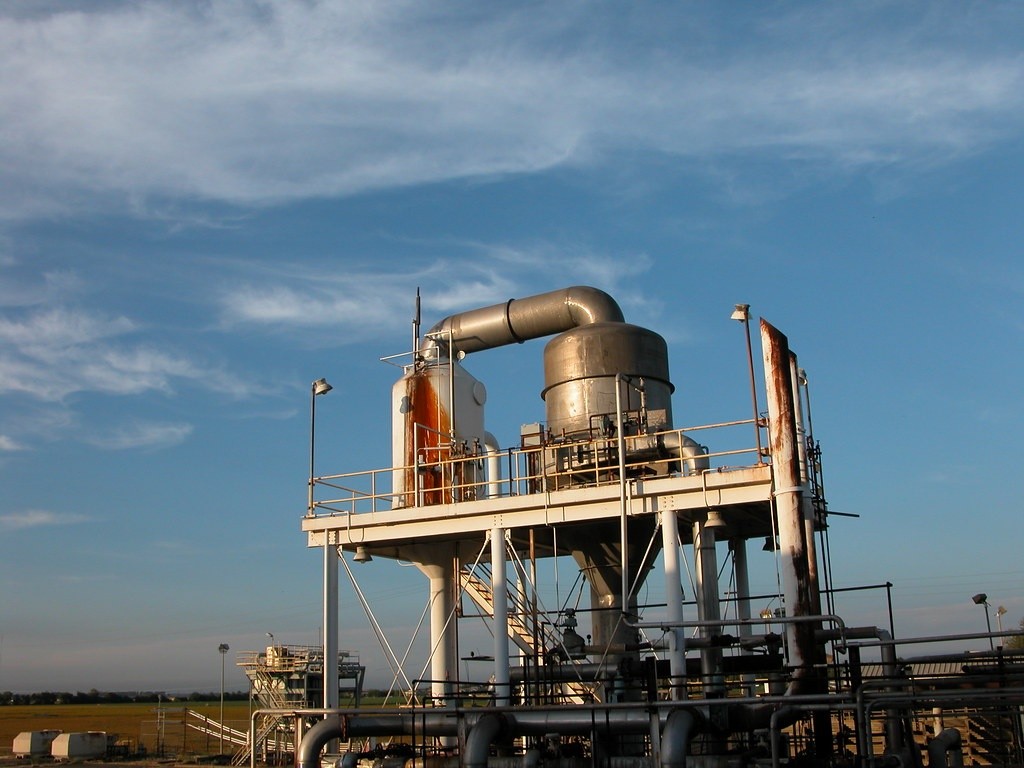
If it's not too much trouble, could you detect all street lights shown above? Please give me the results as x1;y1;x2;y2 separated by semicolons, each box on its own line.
218;643;229;754
